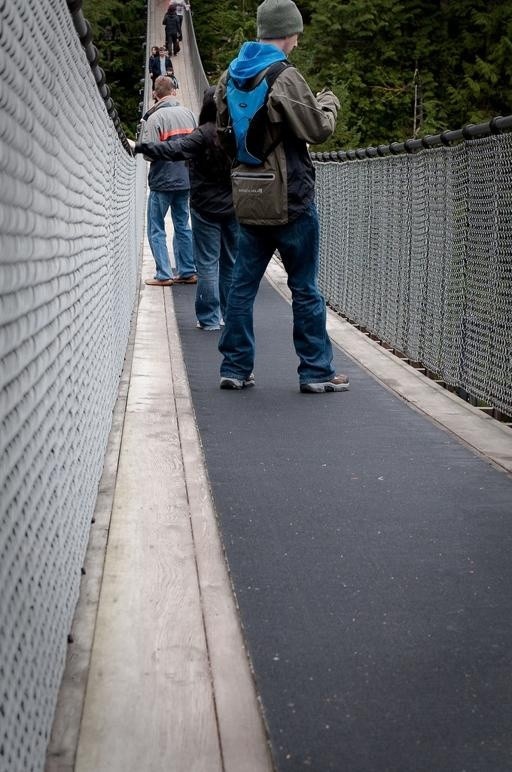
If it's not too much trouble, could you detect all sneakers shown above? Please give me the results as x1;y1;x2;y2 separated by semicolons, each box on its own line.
145;277;174;286
196;316;225;331
173;276;198;283
220;372;255;389
301;374;350;393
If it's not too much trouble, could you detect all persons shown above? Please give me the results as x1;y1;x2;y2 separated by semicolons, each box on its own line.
213;0;350;392
121;1;239;329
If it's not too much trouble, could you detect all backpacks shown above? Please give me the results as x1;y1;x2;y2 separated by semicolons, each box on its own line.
217;62;294;167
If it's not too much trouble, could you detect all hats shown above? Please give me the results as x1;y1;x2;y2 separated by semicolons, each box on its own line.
256;0;303;39
